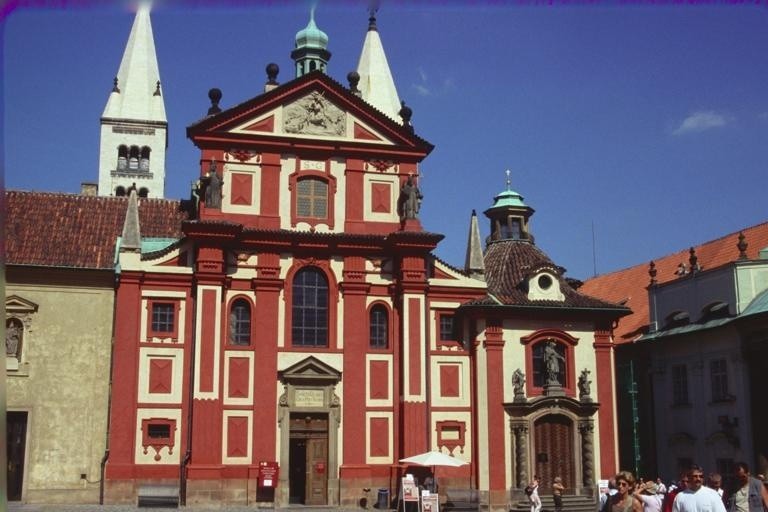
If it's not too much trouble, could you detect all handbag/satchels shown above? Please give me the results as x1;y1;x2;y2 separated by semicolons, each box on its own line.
525;486;532;495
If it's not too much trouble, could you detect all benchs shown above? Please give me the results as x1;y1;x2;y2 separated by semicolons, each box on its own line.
441;489;478;512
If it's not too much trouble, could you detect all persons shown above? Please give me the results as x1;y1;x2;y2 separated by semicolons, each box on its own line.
528;475;542;512
599;461;768;512
544;340;566;384
398;175;423;220
553;477;565;512
204;159;224;208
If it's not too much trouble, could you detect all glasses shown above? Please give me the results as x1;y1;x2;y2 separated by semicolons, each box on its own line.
693;475;704;478
617;482;626;487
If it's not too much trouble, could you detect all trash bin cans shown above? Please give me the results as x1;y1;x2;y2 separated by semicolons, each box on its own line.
379;488;388;510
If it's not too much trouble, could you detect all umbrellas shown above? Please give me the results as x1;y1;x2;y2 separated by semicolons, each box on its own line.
398;451;469;493
